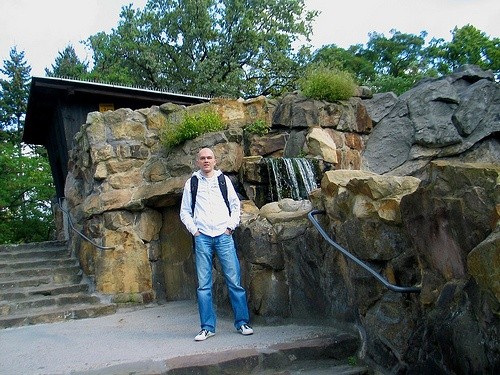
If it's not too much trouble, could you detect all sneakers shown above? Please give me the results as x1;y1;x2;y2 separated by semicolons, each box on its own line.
236;323;254;335
194;328;215;341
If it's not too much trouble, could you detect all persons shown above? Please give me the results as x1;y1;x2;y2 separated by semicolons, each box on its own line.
180;148;254;341
